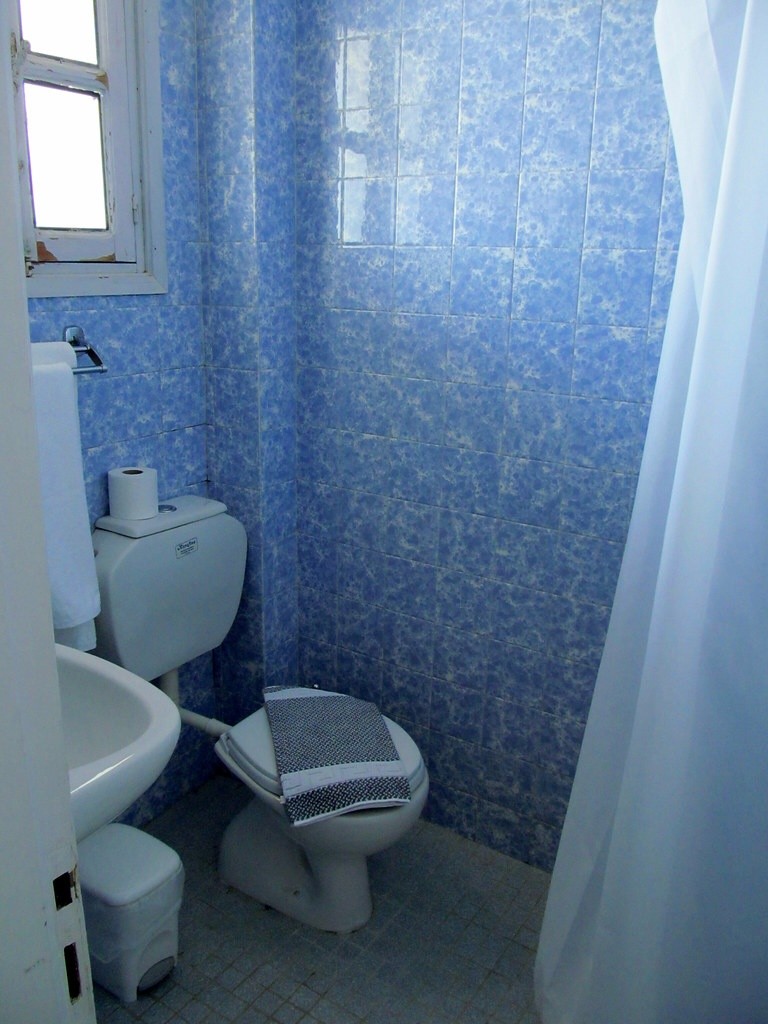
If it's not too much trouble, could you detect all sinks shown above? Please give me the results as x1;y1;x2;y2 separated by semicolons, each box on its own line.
54;640;186;845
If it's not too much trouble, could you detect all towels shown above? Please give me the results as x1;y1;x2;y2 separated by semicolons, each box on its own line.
31;339;78;402
32;360;104;653
260;681;413;830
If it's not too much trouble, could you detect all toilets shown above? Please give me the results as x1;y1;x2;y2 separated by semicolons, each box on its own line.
89;495;429;935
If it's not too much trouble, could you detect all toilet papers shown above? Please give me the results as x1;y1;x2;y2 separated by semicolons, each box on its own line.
107;466;160;521
